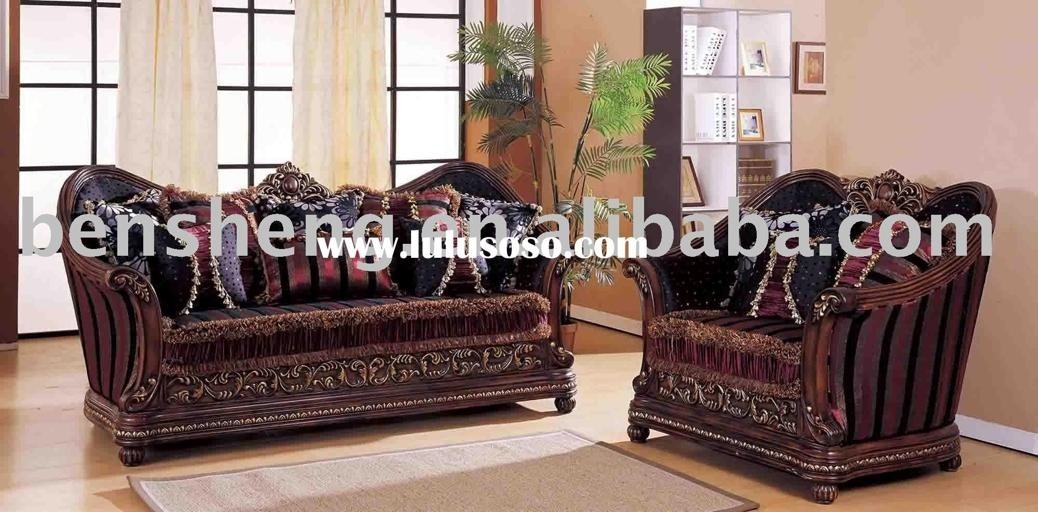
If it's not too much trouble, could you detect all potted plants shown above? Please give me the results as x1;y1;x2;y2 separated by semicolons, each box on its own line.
448;22;674;356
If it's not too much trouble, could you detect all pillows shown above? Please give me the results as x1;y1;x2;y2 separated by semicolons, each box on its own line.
726;209;941;322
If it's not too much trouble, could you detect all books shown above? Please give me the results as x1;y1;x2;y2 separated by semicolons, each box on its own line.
683;24;736;143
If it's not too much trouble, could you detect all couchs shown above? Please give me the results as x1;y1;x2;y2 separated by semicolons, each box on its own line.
54;161;577;465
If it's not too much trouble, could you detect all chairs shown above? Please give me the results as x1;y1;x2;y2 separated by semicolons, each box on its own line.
624;168;999;504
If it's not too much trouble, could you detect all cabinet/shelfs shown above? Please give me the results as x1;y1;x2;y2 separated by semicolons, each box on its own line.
643;6;793;360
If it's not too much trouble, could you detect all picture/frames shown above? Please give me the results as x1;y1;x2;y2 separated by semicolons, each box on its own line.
740;40;772;77
737;109;765;142
795;41;827;95
680;156;706;207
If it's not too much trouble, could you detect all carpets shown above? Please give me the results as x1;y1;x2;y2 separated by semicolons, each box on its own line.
128;428;759;512
